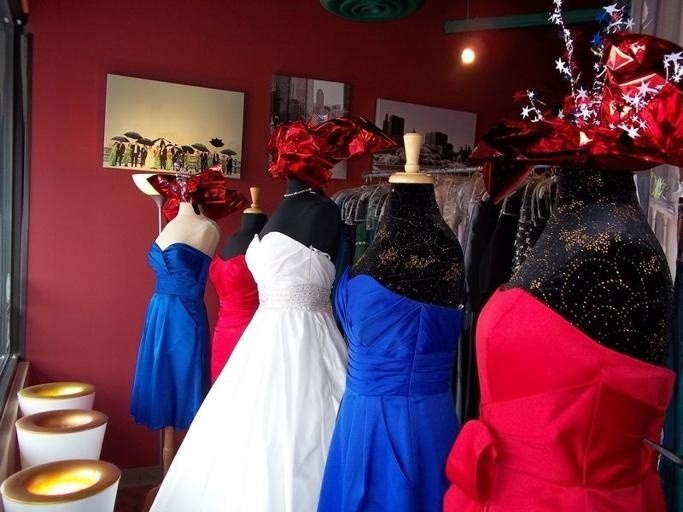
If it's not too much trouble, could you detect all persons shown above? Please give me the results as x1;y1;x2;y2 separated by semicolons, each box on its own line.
443;167;676;512
129;192;220;480
159;145;167;169
116;140;124;166
213;151;219;165
146;145;155;167
208;187;267;382
131;142;140;167
154;145;160;167
123;143;131;167
225;155;233;174
140;145;147;166
201;151;208;172
110;142;118;165
233;159;239;173
146;172;348;511
319;132;467;512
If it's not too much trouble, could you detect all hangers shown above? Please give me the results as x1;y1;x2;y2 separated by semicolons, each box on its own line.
499;160;560;220
339;168;478;223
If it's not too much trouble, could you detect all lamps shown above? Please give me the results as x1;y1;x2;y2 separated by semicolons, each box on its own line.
18;382;96;414
1;460;122;512
16;408;108;470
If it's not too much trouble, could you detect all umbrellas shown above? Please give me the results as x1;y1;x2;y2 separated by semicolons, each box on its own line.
124;132;142;140
181;145;194;154
192;143;208;151
137;138;154;145
111;136;129;143
220;149;236;155
